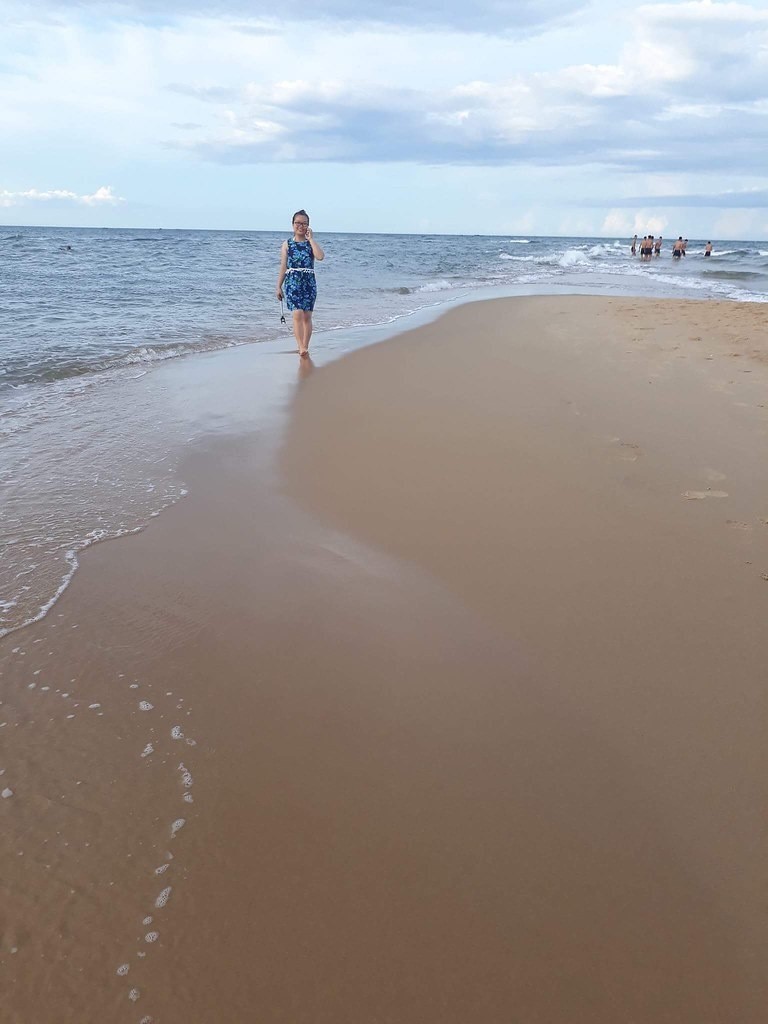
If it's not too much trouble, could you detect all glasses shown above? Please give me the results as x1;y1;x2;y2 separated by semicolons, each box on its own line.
294;221;308;226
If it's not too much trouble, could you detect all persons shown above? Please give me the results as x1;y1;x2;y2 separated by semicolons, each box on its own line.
277;210;324;356
705;242;712;256
631;235;662;261
672;237;688;258
